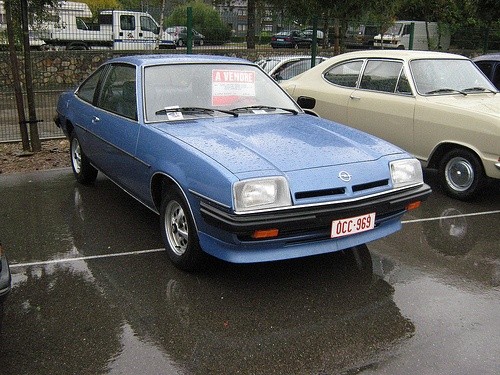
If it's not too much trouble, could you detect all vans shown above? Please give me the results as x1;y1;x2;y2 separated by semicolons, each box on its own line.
372;20;440;51
345;24;380;49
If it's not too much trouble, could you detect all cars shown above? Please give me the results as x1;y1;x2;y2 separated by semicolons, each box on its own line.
275;49;500;201
270;30;318;49
466;53;500;94
306;29;331;48
53;54;431;272
252;55;329;81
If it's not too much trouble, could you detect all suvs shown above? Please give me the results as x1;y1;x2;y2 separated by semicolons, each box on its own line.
166;25;206;48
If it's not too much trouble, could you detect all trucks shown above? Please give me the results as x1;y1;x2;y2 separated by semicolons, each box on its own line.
0;0;177;53
